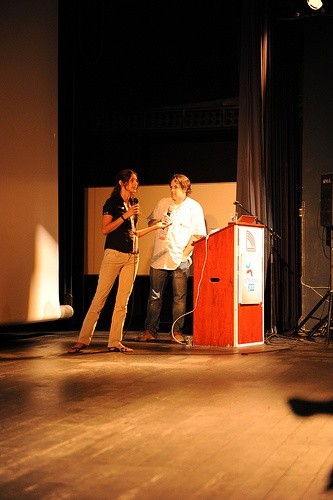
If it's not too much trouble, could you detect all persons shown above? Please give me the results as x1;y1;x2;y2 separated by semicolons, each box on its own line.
135;174;206;342
68;169;170;353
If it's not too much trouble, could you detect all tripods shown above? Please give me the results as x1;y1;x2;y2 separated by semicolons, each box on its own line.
294;228;333;342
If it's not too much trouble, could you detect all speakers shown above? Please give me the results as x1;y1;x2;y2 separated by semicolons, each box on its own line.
320;174;333;227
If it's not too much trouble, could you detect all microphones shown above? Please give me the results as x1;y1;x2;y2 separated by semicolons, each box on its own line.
162;205;173;230
134;197;139;224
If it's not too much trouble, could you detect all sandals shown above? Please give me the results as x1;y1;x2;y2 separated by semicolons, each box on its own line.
68;343;87;353
107;345;134;352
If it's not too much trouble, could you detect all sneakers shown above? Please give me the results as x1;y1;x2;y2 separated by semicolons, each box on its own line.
134;330;158;341
168;331;186;343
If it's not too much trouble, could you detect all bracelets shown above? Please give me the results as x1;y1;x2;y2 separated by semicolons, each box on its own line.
120;215;126;221
